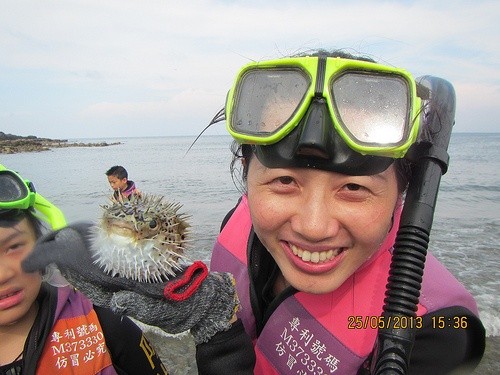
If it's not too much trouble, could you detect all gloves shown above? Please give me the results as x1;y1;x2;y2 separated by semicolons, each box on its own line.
21;222;207;303
52;251;239;345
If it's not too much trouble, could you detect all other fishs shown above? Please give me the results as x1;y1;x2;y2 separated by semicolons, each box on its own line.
84;187;191;290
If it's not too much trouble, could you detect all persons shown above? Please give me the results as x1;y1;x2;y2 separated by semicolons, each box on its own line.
104;164;142;204
0;162;170;375
55;48;487;375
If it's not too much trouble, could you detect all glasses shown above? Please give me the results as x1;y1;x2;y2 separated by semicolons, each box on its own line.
0;164;68;233
225;55;424;161
